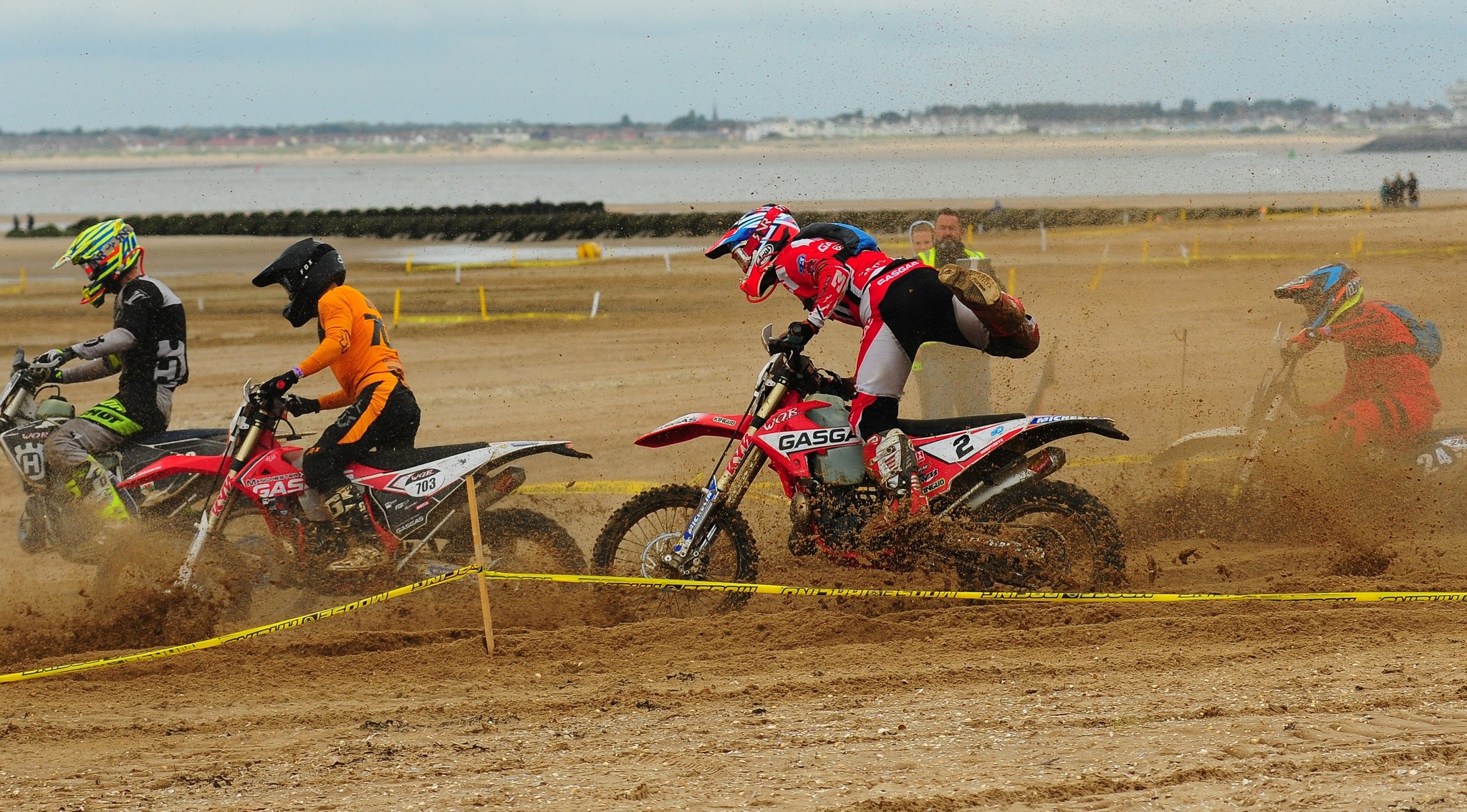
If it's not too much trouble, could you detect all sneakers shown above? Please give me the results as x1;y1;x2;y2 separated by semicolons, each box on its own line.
938;263;1002;306
862;496;929;544
74;529;128;558
327;540;387;571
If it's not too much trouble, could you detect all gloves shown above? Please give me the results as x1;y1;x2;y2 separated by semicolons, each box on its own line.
778;320;817;346
259;370;299;410
38;348;77;367
47;370;63;384
1300;328;1331;344
285;393;315;417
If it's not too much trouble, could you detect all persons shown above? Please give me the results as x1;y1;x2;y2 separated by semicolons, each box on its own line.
250;236;433;569
1380;171;1422;208
35;218;188;564
11;215;20;234
706;203;1040;544
906;206;1008;421
1252;261;1442;534
25;215;35;232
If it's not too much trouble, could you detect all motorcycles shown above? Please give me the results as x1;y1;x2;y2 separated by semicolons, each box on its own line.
1;344;319;590
1148;330;1467;541
88;378;589;625
588;324;1132;628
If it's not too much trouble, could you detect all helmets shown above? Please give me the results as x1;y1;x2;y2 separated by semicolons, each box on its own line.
1273;263;1364;330
252;237;346;328
51;218;141;308
705;203;800;298
